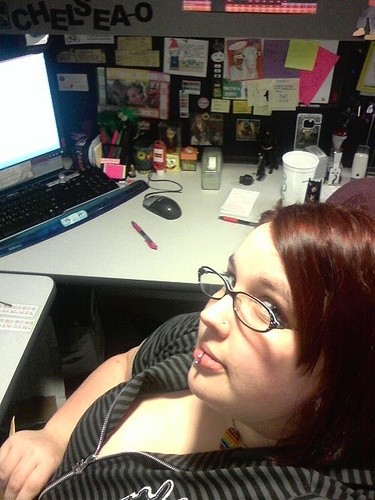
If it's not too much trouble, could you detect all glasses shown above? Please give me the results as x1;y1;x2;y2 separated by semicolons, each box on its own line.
198;266;295;333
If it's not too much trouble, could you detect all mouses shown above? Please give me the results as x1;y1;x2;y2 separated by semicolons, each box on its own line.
143;195;181;219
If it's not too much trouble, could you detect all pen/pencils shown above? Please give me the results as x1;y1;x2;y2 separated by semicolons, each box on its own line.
218;216;258;228
100;129;123;158
131;221;158;250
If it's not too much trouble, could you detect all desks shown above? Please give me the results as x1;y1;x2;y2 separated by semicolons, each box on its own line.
0;163;281;427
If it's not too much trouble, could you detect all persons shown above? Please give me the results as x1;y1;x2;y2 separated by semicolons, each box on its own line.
124;38;264;147
0;202;375;500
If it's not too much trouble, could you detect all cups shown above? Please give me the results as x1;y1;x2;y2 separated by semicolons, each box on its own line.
280;151;320;208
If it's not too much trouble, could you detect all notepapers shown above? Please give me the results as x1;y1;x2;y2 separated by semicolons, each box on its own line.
107;164;126;179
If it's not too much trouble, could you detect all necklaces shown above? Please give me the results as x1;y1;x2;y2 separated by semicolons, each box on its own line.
219;418;248;450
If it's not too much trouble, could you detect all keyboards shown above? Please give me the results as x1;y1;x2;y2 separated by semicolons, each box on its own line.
0;166;119;240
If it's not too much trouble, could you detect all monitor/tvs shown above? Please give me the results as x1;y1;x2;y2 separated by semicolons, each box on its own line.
0;41;67;178
